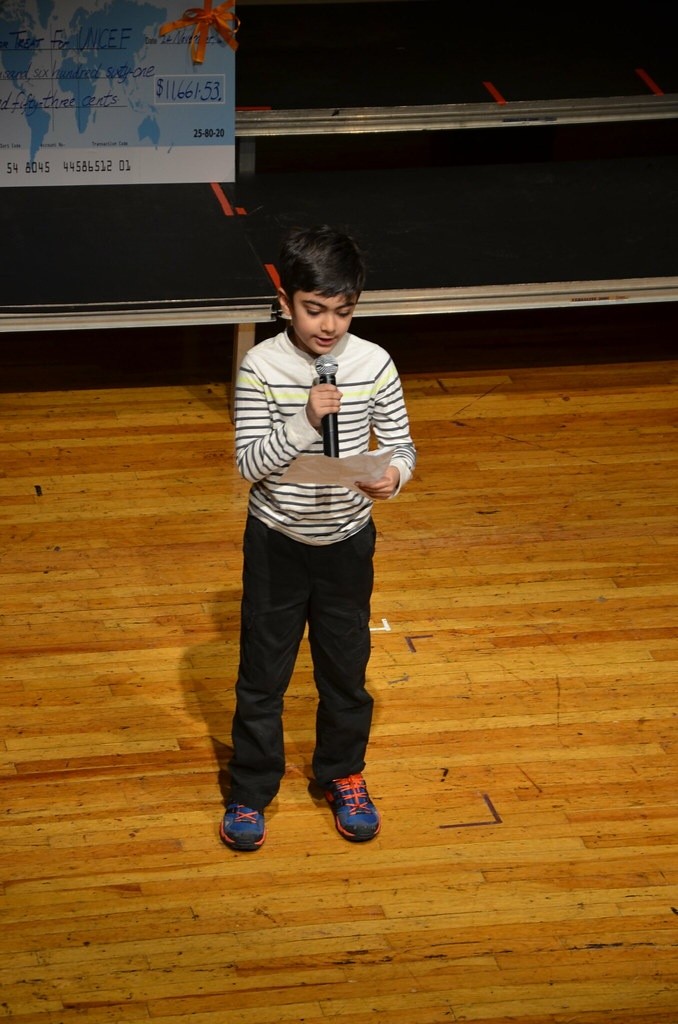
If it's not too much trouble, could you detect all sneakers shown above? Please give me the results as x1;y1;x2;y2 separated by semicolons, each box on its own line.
325;771;382;841
219;798;267;850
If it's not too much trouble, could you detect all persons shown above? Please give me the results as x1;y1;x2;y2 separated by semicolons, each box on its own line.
220;232;416;851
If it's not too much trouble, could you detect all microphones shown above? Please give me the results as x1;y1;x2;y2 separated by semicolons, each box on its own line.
314;353;340;459
